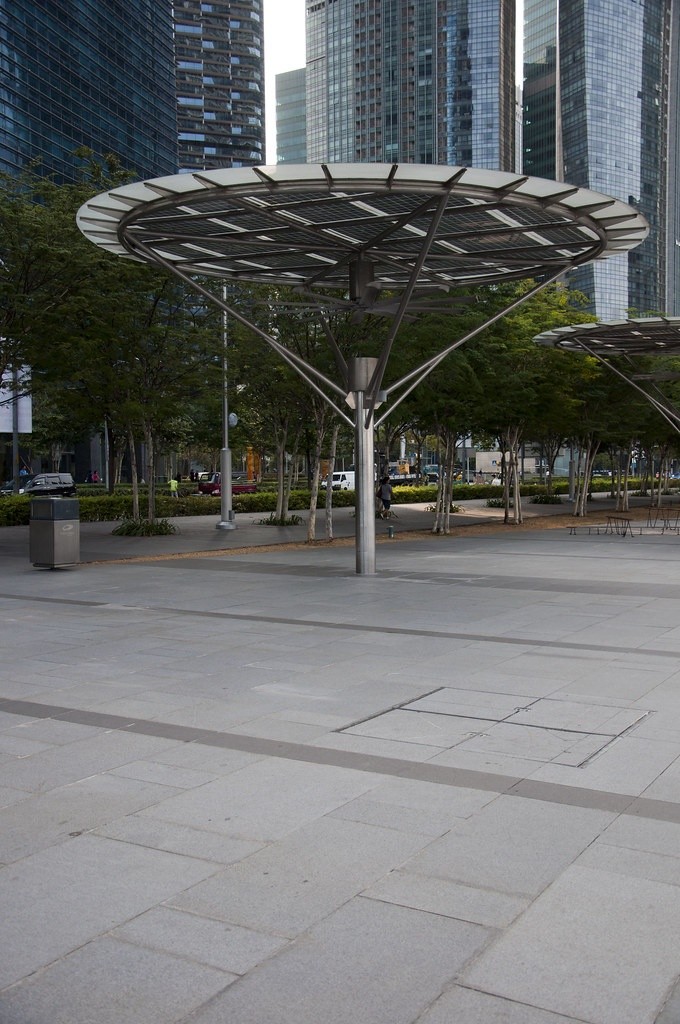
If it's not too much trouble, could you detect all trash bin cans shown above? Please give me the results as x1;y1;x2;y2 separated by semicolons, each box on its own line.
30;497;81;569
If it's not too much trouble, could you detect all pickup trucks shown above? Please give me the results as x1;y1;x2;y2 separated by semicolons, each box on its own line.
198;472;256;495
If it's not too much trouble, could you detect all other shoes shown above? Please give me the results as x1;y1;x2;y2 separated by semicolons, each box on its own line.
386;517;389;520
380;513;384;520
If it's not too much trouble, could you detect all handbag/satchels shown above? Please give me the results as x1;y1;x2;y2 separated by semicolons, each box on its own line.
376;486;383;499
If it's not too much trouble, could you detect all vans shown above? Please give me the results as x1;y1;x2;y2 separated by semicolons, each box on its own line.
0;473;77;498
321;471;355;490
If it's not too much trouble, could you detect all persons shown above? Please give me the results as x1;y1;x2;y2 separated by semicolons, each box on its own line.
20;466;28;475
86;470;100;483
491;475;496;485
190;469;199;482
379;477;396;519
168;477;178;498
